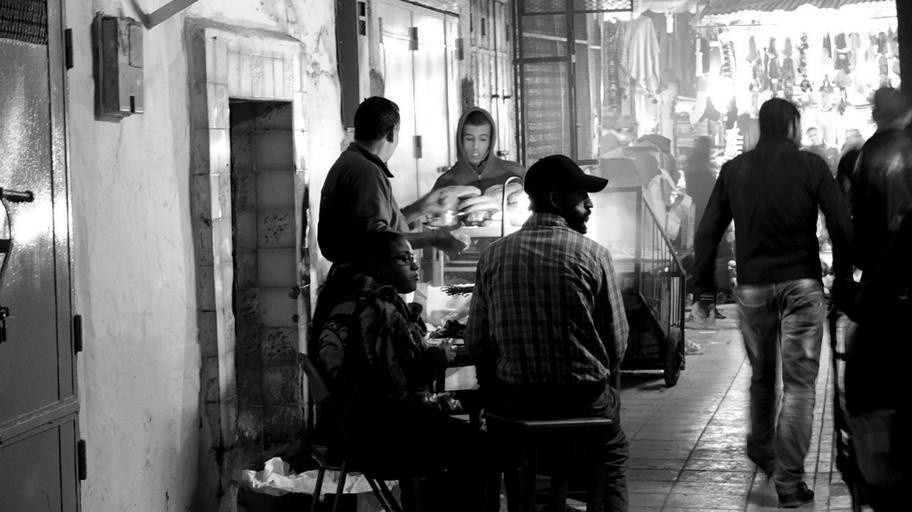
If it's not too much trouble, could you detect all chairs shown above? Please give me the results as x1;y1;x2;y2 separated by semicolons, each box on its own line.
297;352;401;512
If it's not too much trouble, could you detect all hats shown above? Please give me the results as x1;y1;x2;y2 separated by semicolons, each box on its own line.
524;154;608;194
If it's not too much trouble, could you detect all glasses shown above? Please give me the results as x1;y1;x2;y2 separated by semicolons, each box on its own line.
391;249;415;267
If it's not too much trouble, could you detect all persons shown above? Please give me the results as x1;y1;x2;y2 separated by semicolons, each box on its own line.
690;97;854;509
433;105;524;187
636;135;681;185
802;127;841;251
318;90;468;263
465;154;631;509
840;130;866;155
684;135;727;319
831;83;911;443
307;228;503;510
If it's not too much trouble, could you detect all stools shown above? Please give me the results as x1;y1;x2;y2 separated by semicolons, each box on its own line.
483;412;613;512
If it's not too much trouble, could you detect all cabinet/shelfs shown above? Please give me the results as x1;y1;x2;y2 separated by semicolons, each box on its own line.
405;225;519;433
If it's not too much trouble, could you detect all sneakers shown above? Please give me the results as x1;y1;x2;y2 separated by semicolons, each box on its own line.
778;484;813;508
747;433;775;474
